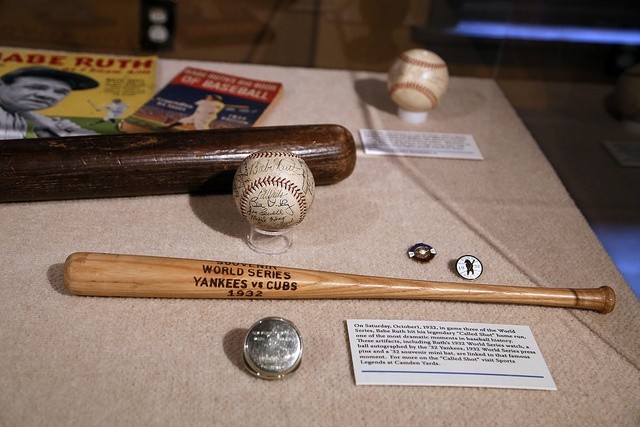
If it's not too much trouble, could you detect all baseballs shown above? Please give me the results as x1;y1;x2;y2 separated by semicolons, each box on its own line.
386;49;448;112
233;149;315;231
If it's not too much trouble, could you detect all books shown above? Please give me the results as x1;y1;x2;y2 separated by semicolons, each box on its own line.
0;47;161;137
120;66;284;136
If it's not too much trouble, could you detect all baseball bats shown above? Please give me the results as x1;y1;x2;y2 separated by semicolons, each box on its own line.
87;99;101;113
62;252;616;314
223;105;249;109
1;124;356;202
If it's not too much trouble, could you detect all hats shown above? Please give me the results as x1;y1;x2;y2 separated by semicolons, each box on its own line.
2;66;99;90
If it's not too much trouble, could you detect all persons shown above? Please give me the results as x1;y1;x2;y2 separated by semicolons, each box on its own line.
164;92;226;131
90;99;124;130
0;65;99;140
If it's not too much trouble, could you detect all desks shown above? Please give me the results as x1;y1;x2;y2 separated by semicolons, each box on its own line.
1;46;640;424
